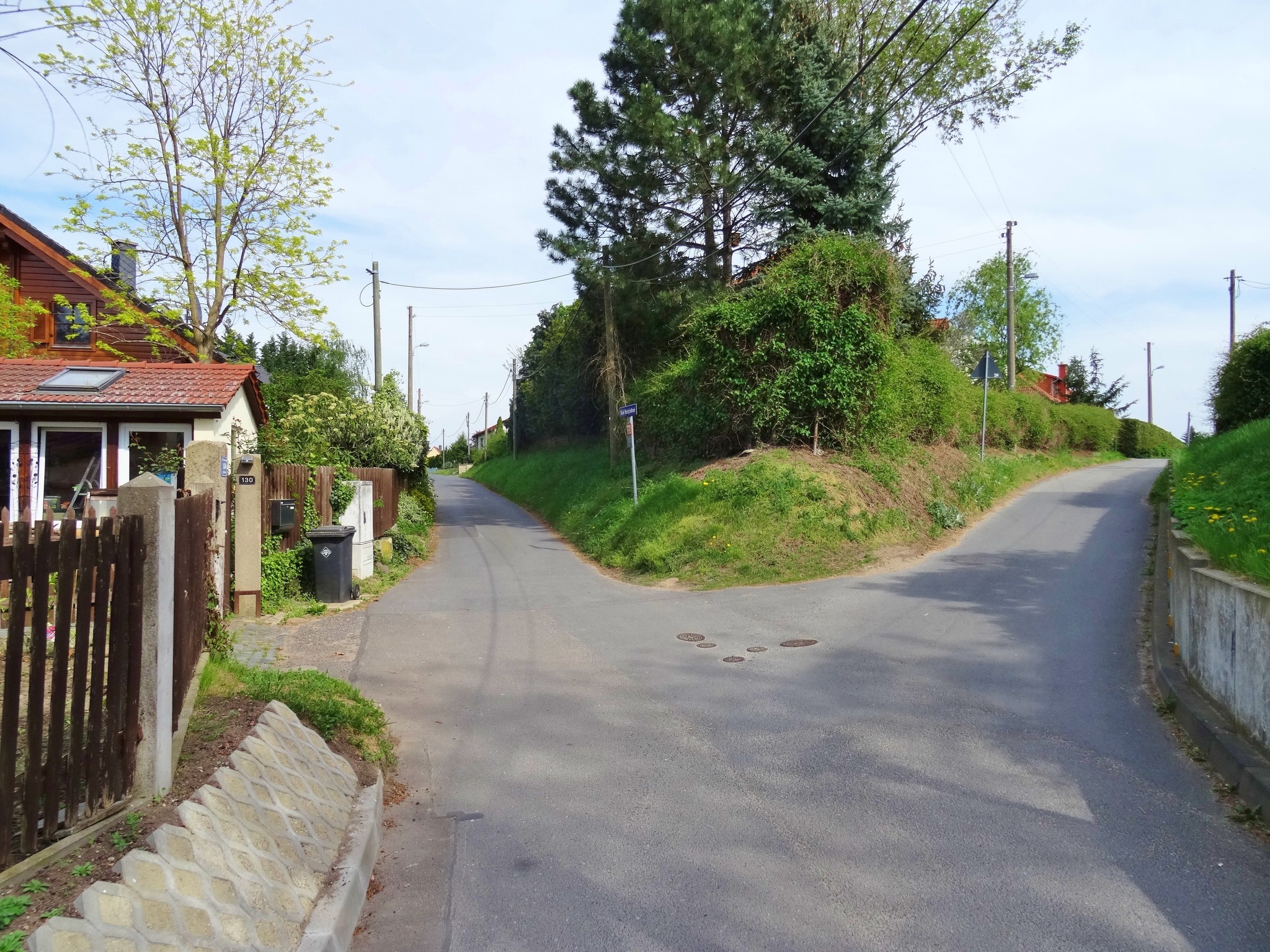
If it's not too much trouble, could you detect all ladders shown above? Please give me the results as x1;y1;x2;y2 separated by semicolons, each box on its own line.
63;451;101;518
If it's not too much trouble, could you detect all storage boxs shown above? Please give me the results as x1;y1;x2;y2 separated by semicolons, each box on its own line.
45;495;62;509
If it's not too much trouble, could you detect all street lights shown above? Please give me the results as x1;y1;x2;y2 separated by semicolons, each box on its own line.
417;400;430;422
1147;364;1165;424
407;342;430;411
1006;272;1040;391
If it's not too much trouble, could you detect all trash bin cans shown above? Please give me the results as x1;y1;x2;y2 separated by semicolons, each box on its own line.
305;526;360;603
